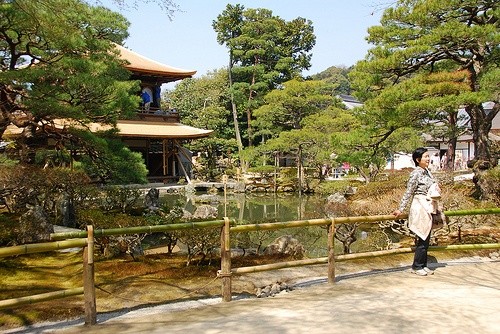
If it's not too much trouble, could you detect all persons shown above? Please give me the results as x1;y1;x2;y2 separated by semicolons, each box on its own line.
430;152;462;173
139;87;153;114
153;82;161;110
390;148;442;276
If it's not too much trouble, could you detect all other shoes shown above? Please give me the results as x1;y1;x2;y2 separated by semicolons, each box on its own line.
424;267;434;275
411;268;427;276
